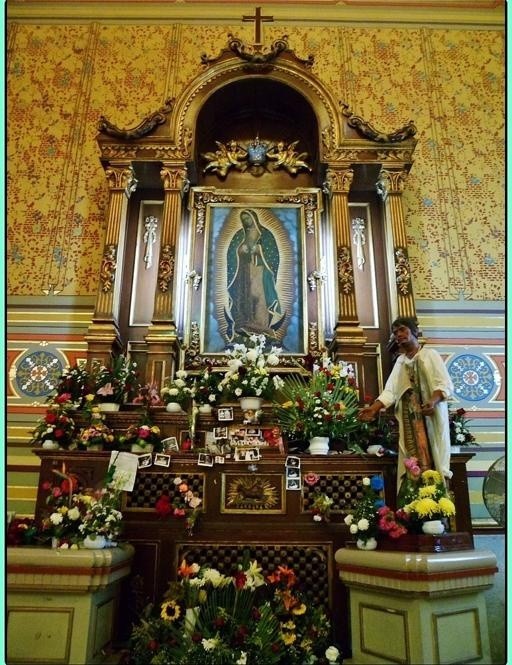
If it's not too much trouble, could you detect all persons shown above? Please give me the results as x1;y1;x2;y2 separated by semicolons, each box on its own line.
358;318;453;492
223;208;285;343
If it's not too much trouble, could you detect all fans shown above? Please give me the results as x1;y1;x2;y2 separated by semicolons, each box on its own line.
483;456;506;531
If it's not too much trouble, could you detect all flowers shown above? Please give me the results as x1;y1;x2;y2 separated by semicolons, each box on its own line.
8;466;125;551
340;455;458;552
46;335;270;415
29;413;160;462
153;480;205;536
270;366;396;453
127;556;344;664
305;470;333;527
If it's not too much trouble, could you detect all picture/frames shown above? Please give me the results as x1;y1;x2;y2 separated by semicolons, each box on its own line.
178;187;323;377
